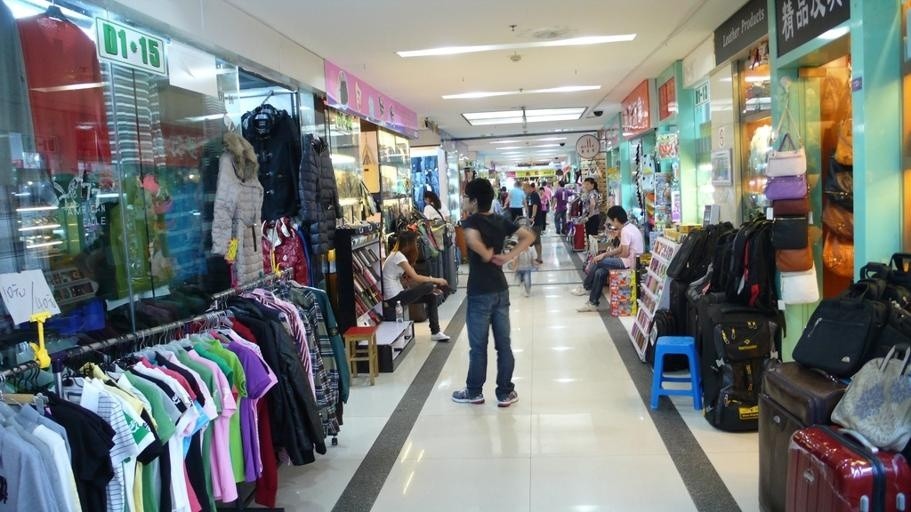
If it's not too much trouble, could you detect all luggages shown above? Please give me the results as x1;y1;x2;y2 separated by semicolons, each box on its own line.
788;420;910;511
758;361;846;512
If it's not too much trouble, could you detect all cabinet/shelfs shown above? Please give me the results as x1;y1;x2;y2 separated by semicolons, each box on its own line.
377;130;412;216
588;234;610;256
352;240;385;340
627;236;681;362
356;320;415;373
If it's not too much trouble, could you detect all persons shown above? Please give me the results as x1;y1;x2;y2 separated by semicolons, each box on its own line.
581;178;601;254
512;226;543;298
570;205;643;313
382;231;451;340
423;191;450;223
452;178;536;407
499;180;576;264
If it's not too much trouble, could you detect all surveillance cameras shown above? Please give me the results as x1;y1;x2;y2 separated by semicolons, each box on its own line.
593;111;603;117
555;157;558;159
560;143;565;147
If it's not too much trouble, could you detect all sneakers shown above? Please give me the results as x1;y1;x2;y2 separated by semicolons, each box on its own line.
576;301;599;312
452;390;484;404
497;391;519;407
570;285;588;296
431;332;450;341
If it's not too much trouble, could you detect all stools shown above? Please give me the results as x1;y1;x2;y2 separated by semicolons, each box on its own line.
344;326;378;385
650;335;702;411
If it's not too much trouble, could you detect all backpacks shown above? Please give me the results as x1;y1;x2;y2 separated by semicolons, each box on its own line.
666;220;777;305
699;302;781;432
648;309;688;371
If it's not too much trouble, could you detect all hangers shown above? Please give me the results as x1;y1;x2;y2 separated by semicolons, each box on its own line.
254;103;275;131
1;271;291;407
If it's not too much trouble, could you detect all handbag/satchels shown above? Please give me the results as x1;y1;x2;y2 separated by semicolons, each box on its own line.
766;107;820;305
821;77;854;278
831;346;910;453
793;253;911;376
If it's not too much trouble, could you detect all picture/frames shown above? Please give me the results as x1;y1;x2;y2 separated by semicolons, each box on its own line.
710;148;733;187
